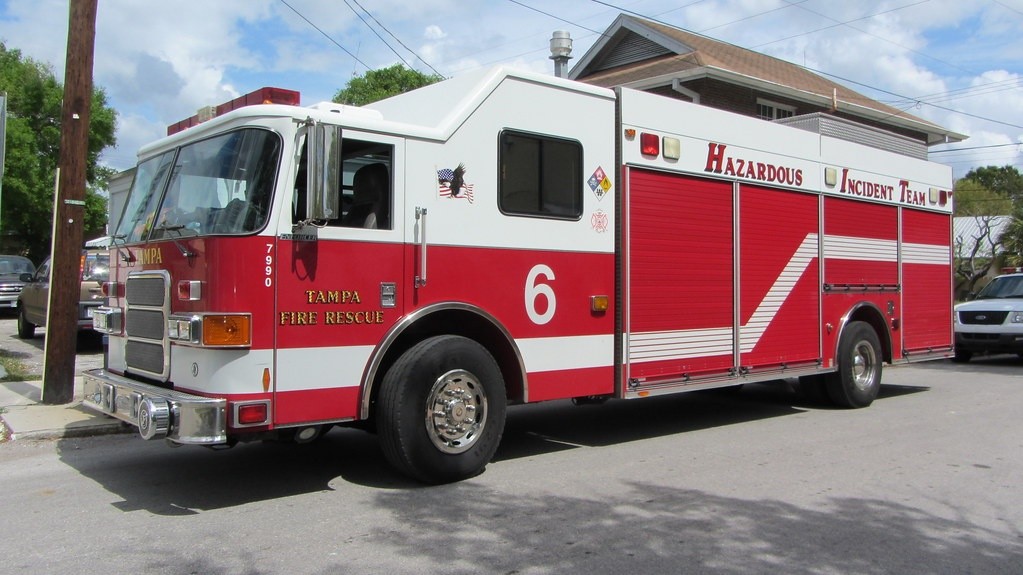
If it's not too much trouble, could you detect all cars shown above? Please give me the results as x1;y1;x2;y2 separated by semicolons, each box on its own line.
0;254;37;314
953;266;1023;363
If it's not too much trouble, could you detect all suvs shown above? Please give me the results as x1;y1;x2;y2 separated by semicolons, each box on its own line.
15;250;111;348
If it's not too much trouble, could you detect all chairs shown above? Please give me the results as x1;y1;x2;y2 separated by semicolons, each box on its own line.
340;162;390;229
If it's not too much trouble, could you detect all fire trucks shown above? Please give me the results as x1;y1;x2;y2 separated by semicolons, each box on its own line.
82;29;958;488
